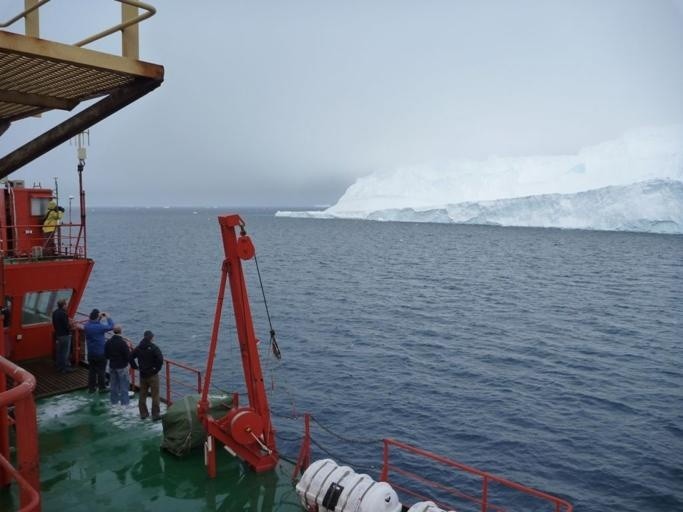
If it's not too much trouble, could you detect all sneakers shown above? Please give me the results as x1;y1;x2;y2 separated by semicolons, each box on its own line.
88;389;134;409
141;413;163;422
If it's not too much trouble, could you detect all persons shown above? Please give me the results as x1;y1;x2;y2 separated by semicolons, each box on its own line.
40;201;64;257
126;330;163;421
51;298;77;374
103;323;134;409
129;434;164;502
82;309;114;394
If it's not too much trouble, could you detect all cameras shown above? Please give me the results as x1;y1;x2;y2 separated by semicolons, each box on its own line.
58;206;62;210
101;312;106;318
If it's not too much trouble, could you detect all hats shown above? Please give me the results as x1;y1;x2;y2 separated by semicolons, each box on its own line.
90;308;100;319
144;331;153;337
113;325;122;332
